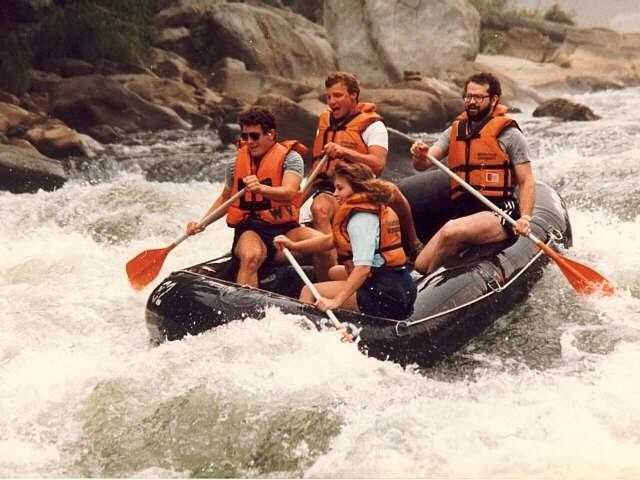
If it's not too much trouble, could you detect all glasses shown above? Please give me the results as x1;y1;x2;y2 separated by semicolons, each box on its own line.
462;93;493;105
240;132;262;142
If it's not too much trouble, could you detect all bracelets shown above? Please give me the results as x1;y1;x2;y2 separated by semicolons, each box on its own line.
521;215;532;222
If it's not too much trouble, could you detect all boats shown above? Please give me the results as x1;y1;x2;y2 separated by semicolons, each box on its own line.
147;168;572;368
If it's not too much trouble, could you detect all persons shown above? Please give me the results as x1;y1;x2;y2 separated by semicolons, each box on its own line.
273;163;417;321
409;72;536;275
302;73;389;235
186;106;338;289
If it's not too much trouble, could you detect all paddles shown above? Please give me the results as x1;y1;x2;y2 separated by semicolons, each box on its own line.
126;186;246;292
418;149;614;297
